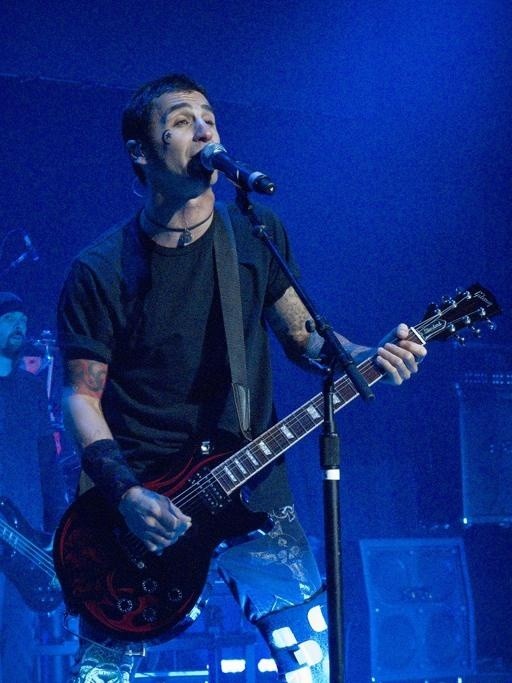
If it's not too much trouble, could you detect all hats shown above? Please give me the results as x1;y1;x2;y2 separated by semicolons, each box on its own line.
0;291;30;318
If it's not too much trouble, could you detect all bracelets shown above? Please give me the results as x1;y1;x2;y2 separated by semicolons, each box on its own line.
79;437;144;509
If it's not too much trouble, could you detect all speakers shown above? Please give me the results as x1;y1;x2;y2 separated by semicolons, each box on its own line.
413;384;511;535
337;534;478;683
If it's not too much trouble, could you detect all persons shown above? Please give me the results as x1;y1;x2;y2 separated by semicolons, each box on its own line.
0;291;83;682
60;72;333;682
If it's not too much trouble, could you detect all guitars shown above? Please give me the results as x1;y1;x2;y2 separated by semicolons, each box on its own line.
56;283;501;641
0;497;64;612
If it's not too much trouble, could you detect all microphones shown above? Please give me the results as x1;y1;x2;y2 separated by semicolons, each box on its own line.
23;232;40;261
201;142;277;195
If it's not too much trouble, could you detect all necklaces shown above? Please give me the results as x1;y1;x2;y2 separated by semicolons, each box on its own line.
142;206;215;244
